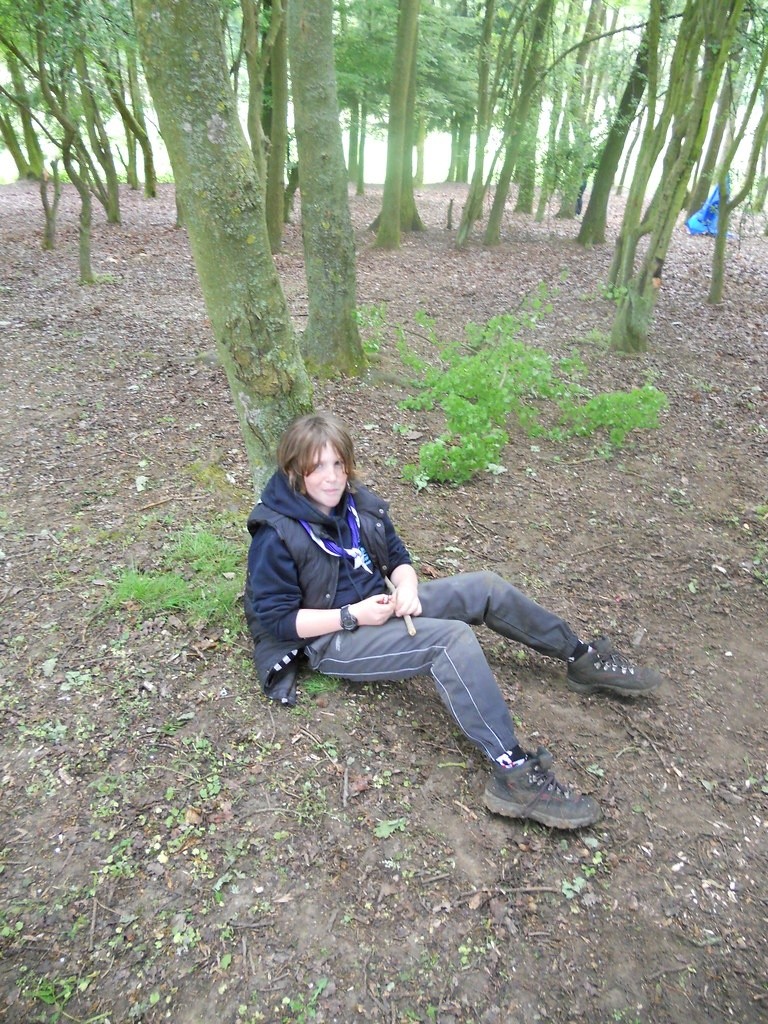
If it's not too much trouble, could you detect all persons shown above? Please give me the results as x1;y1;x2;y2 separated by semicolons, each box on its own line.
242;409;668;831
573;136;594;216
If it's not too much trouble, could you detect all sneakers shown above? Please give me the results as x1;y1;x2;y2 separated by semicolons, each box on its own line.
483;746;603;829
567;634;663;698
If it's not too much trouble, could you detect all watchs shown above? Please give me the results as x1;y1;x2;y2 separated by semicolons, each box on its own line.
338;604;360;633
